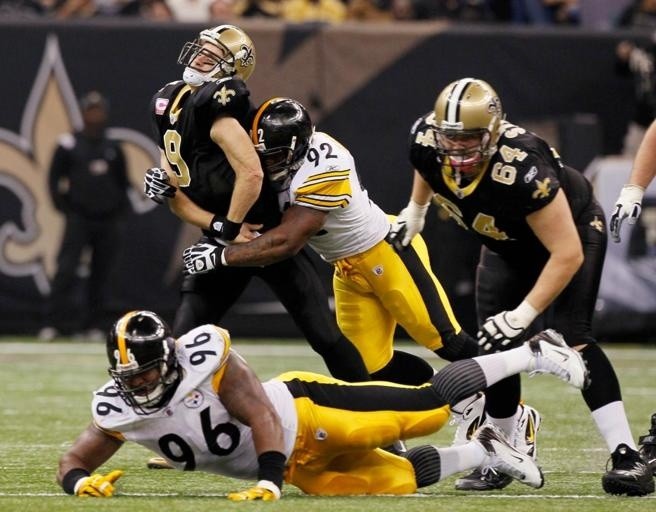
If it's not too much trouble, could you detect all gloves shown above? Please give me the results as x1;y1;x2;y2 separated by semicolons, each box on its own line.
180;241;229;279
226;478;282;503
387;196;431;249
475;299;541;353
607;182;645;245
142;167;178;206
72;468;123;499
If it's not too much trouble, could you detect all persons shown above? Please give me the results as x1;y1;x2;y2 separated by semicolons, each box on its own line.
609;121;656;475
385;75;654;496
1;1;655;33
144;99;540;462
57;310;590;503
153;23;405;454
35;92;129;342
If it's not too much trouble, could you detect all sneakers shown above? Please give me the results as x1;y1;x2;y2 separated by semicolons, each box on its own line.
637;413;656;477
514;402;542;463
448;389;486;447
601;441;656;497
470;422;545;489
523;328;590;391
453;464;514;492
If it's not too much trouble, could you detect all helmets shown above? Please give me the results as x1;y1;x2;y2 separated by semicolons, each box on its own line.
104;307;179;384
434;77;504;158
199;22;257;84
248;95;313;166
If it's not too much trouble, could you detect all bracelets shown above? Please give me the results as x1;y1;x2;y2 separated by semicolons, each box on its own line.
221;247;229;266
60;467;90;496
210;214;241;241
256;450;285;491
